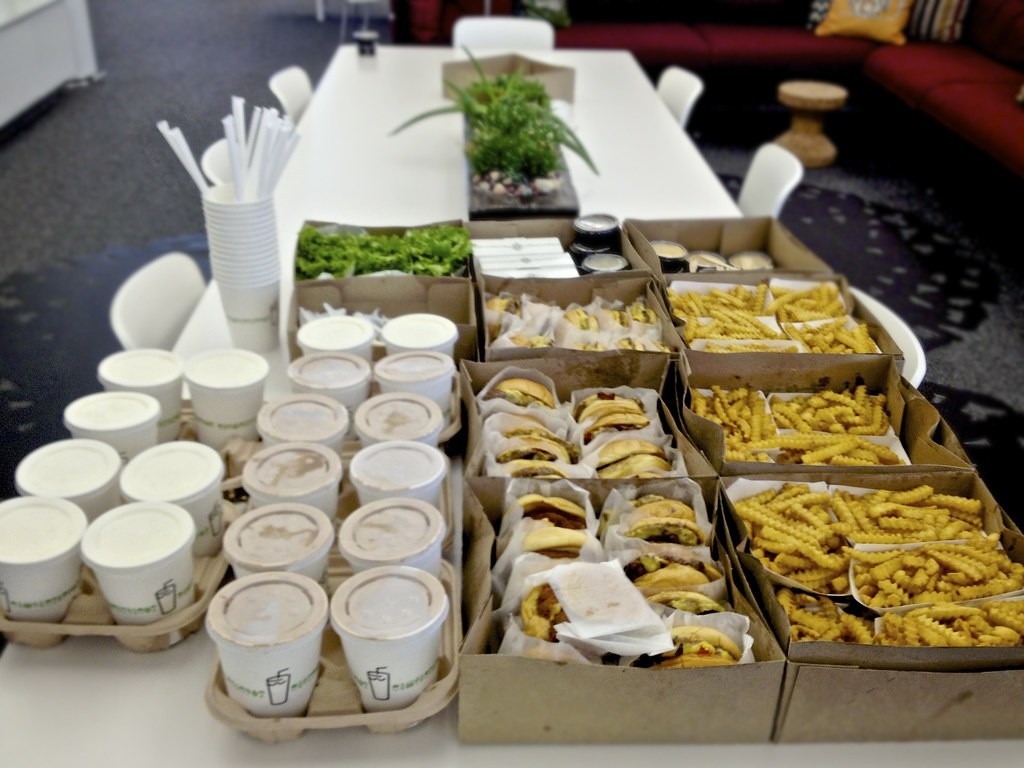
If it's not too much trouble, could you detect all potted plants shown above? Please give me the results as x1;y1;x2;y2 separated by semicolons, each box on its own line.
387;43;600;216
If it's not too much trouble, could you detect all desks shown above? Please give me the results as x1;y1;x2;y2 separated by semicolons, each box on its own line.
0;43;1024;768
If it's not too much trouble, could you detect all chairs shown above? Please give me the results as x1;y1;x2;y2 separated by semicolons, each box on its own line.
654;66;705;130
107;249;206;352
451;16;556;55
738;143;805;218
267;64;312;116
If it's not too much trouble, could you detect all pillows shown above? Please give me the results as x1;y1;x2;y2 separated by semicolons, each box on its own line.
814;0;913;48
908;0;970;42
806;0;829;30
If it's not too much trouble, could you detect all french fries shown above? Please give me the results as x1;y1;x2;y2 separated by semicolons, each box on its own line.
731;482;1024;647
666;281;880;354
686;385;910;467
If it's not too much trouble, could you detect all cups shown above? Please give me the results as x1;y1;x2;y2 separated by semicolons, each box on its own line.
1;190;459;716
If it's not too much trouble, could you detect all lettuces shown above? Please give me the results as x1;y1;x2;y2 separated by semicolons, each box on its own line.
296;222;473;277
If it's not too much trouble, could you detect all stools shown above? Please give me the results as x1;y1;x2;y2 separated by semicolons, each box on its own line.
769;81;846;169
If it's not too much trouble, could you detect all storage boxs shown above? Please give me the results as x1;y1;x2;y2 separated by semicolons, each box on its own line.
289;215;482;363
456;220;1024;743
440;53;575;107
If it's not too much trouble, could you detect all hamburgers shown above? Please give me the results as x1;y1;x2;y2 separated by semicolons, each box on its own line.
482;296;749;669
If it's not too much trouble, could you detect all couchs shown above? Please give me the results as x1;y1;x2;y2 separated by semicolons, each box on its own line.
390;0;1024;217
